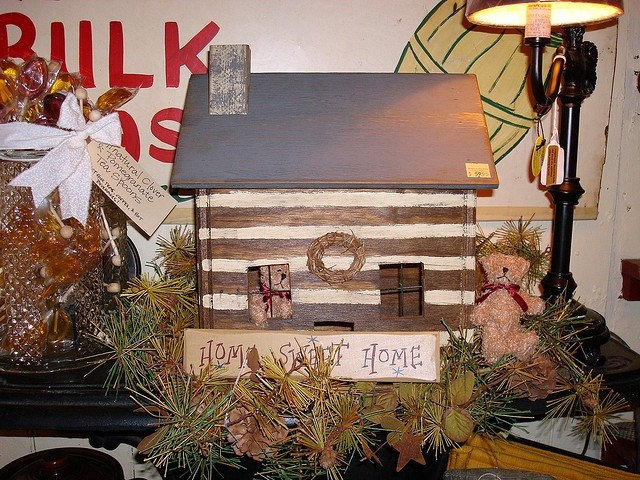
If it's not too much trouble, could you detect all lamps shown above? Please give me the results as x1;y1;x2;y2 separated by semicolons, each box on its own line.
463;1;624;344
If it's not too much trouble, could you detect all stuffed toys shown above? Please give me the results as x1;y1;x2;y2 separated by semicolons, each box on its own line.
249;264;292;325
469;253;545;366
